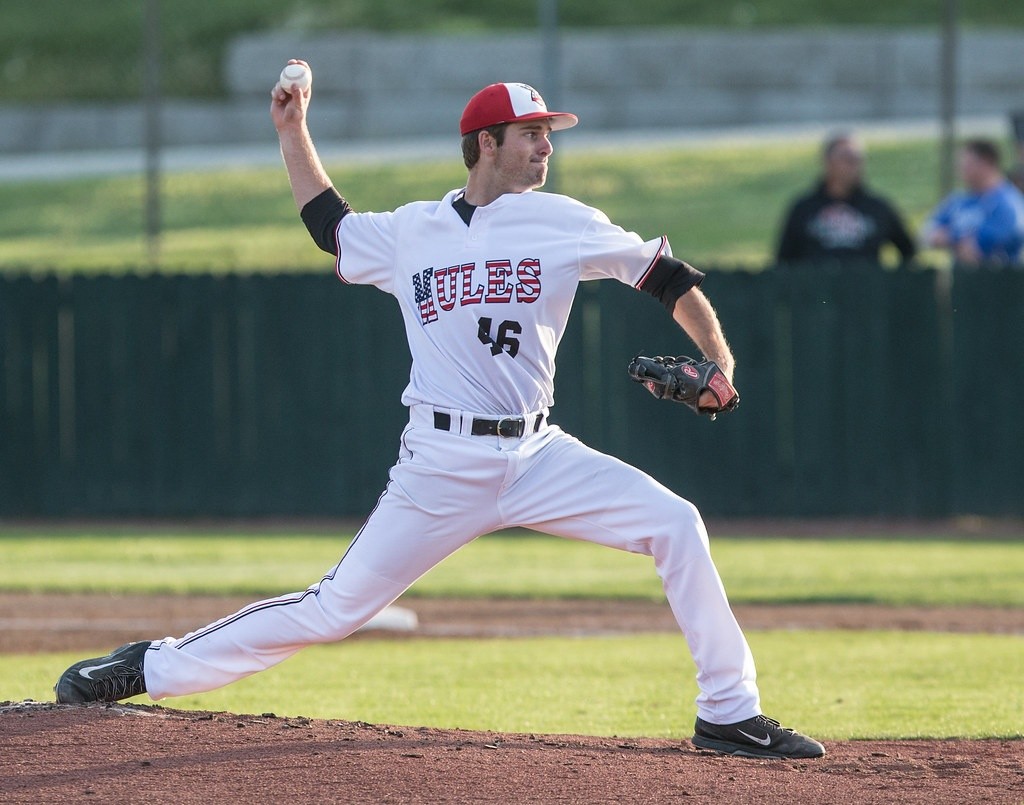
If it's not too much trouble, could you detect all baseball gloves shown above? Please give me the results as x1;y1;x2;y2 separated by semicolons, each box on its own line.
625;348;741;423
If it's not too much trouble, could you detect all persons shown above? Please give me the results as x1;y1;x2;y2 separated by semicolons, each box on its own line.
775;132;916;267
918;137;1024;267
55;58;826;760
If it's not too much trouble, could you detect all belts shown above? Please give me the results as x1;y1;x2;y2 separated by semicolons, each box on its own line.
434;411;549;438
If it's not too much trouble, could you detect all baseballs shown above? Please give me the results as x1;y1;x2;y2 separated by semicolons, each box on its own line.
277;62;313;97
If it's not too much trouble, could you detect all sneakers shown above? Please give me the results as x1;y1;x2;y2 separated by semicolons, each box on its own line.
52;641;152;704
691;712;825;759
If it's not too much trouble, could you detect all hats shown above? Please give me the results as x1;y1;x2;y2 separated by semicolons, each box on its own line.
459;82;578;137
